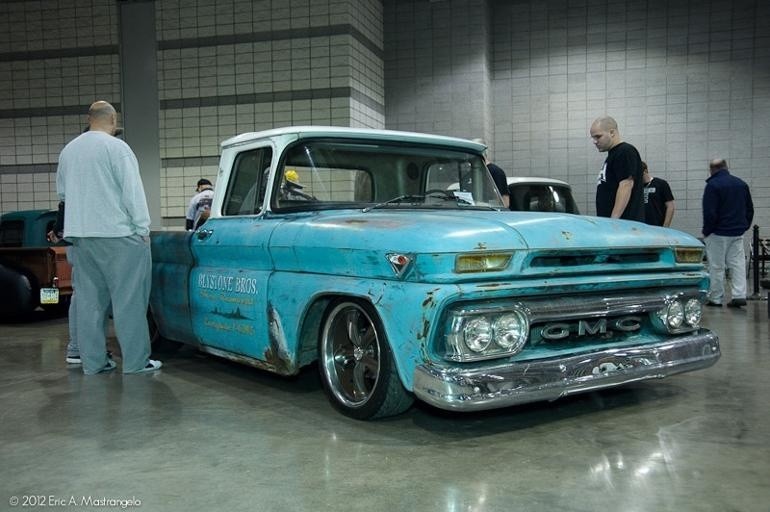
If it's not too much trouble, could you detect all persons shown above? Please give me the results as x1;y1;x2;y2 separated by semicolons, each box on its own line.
48;123;126;364
186;179;215;234
461;138;512;213
54;101;165;377
702;158;755;307
282;169;305;202
638;160;675;231
591;115;649;225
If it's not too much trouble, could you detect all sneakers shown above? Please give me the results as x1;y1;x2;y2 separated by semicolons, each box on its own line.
102;360;117;371
726;297;748;308
138;358;164;371
704;301;723;309
65;355;82;364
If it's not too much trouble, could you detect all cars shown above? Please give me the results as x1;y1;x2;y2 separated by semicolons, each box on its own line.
0;207;90;319
444;177;583;217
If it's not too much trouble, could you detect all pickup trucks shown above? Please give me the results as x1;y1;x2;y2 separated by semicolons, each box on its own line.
142;126;726;416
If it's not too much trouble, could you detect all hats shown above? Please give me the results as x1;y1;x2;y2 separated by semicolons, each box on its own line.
194;179;212;192
284;170;299;183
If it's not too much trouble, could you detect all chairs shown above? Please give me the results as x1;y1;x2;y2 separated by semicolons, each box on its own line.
747;232;770;279
